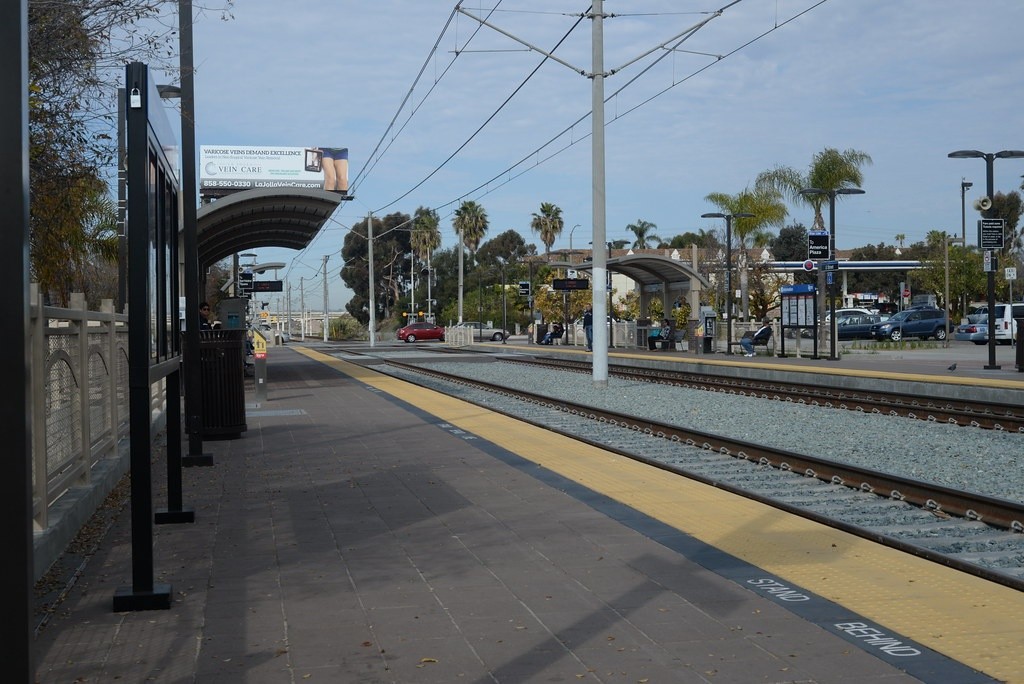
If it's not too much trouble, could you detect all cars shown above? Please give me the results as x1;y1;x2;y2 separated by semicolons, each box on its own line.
453;322;510;342
954;314;988;345
818;314;891;341
395;322;445;343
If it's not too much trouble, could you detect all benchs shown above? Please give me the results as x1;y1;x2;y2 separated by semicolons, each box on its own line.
653;329;687;352
727;330;774;356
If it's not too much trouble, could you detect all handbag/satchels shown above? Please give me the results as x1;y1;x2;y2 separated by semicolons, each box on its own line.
246;355;255;365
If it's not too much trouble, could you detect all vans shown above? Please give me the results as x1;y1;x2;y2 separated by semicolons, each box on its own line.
987;303;1024;345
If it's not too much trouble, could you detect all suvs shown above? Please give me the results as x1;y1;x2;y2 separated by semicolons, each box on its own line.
965;305;989;324
869;305;955;342
801;308;873;339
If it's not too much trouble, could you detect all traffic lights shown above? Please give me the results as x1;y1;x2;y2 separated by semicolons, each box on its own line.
803;260;814;272
421;268;429;276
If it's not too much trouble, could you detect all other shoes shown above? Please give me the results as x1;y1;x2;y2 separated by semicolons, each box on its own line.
586;348;591;352
753;353;757;356
744;353;753;358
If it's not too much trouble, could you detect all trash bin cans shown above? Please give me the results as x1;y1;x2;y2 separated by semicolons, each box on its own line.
180;328;247;440
537;323;548;342
1014;319;1024;373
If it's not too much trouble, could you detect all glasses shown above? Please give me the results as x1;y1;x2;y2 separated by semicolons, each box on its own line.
203;308;210;312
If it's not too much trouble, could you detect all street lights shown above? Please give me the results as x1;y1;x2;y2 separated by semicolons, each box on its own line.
588;240;631;348
701;212;756;355
947;150;1024;370
797;187;866;361
961;181;973;319
569;224;582;264
116;83;182;318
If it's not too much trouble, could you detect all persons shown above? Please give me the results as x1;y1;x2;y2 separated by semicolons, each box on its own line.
536;322;564;345
740;317;772;356
648;319;671;352
318;148;348;190
199;302;210;329
583;303;593;351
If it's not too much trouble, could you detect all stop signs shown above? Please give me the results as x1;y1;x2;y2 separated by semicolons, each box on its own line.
902;289;911;298
